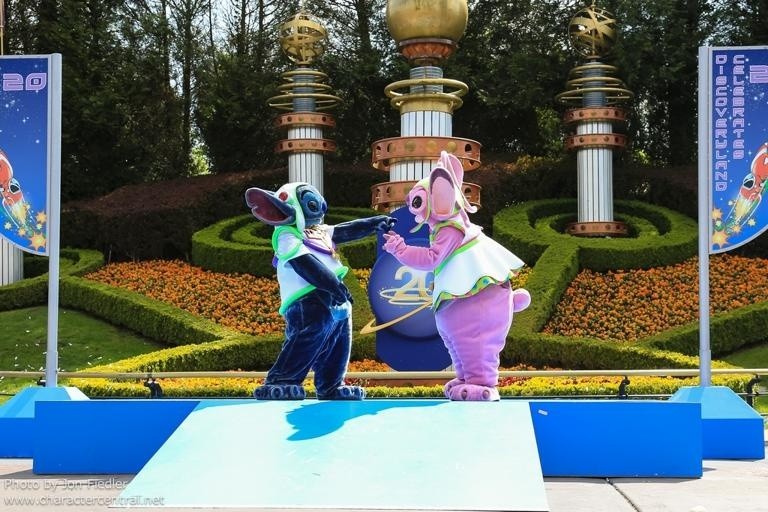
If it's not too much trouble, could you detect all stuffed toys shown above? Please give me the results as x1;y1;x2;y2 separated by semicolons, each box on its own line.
244;181;397;399
381;151;532;402
